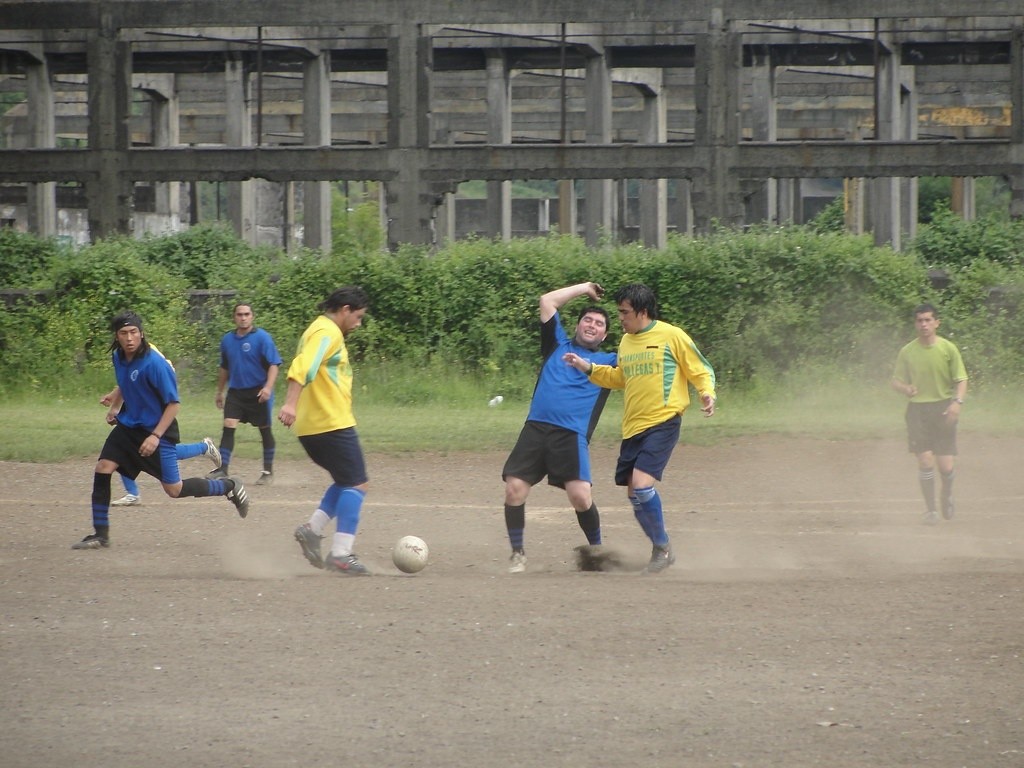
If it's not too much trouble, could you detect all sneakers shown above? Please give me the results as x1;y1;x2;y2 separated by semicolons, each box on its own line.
509;553;527;573
648;537;676;573
324;550;370;575
72;534;110;549
227;477;250;518
924;510;939;525
942;498;955;520
293;523;326;569
111;494;141;506
206;467;229;480
256;471;273;484
200;437;222;467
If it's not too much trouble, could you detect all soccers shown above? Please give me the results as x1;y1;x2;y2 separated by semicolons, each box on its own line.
393;535;429;573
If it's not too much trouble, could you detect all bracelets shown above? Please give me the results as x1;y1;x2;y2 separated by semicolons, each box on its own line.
953;398;962;406
584;363;593;377
151;432;160;440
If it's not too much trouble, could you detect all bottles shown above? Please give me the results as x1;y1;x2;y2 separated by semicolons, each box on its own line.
488;395;503;406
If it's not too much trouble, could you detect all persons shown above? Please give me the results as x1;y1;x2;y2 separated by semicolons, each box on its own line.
893;304;968;526
562;284;717;572
503;282;618;575
278;289;370;575
69;313;249;550
206;306;281;485
